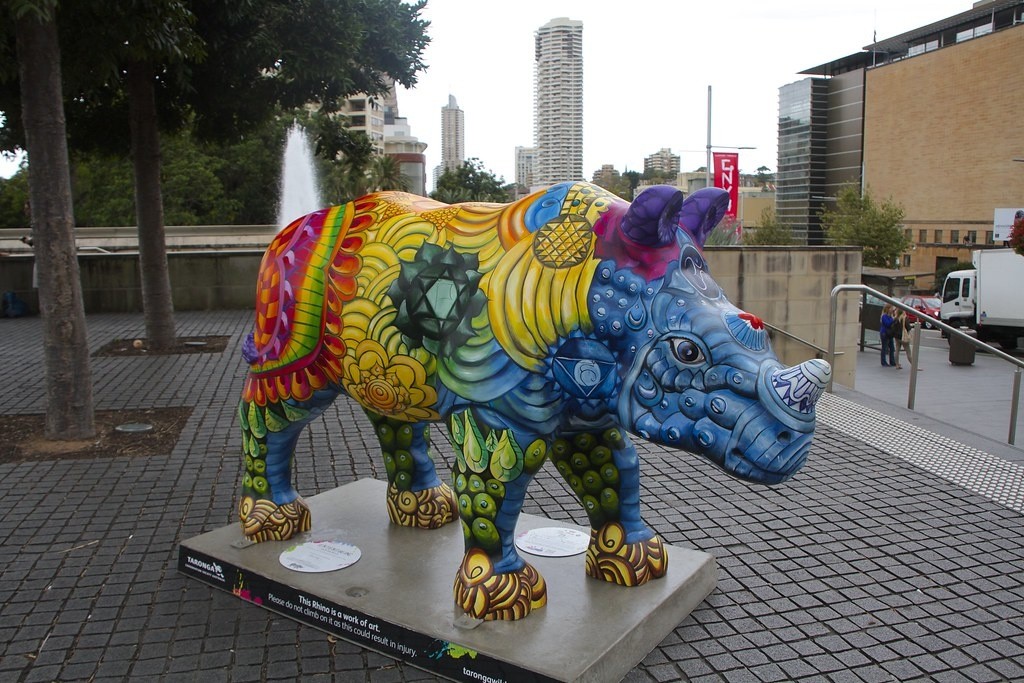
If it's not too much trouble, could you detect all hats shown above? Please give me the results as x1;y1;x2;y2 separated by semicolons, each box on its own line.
1015;210;1024;218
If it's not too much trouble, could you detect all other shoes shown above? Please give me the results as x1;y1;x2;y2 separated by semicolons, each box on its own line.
917;368;924;371
892;363;896;366
885;364;890;367
896;366;903;370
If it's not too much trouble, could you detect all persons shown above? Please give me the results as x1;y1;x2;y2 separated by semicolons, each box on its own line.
880;302;924;371
20;201;39;288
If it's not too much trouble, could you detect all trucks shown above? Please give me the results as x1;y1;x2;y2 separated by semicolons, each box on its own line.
940;247;1024;350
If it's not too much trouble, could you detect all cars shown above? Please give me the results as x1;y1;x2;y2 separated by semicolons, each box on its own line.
897;295;940;329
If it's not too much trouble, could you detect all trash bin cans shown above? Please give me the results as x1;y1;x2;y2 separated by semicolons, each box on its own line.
949;326;977;366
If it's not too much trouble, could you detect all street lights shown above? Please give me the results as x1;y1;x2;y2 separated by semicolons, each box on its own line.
702;145;757;191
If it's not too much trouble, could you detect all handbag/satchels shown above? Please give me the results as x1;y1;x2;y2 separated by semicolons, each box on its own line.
886;327;898;338
902;329;913;343
